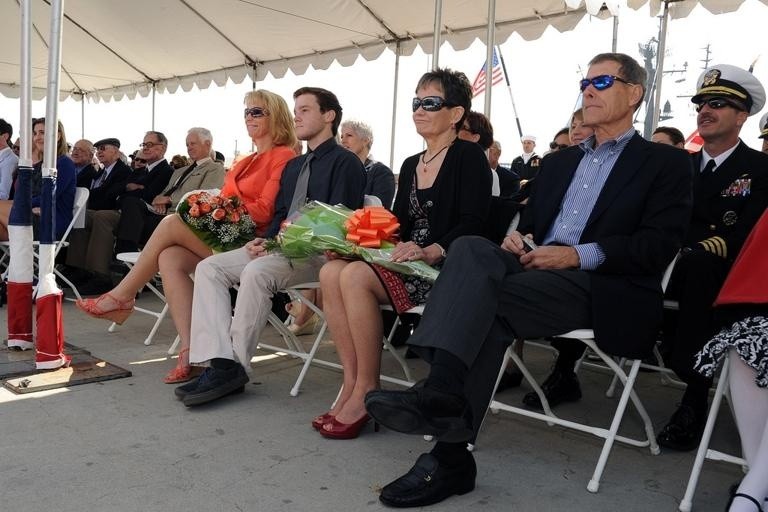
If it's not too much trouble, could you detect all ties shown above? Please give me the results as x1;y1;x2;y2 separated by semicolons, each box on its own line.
286;153;315;220
700;160;716;184
164;162;197;196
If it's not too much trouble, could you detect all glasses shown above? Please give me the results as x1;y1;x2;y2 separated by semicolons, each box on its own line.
412;96;449;113
139;142;164;149
244;107;266;118
549;142;569;150
694;97;745;112
579;74;635;94
135;156;147;164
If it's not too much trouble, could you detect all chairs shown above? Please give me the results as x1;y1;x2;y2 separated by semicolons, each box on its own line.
468;248;684;495
678;356;749;512
331;302;434;442
0;187;90;303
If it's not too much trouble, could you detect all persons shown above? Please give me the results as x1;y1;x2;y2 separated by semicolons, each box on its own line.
692;211;768;511
174;86;367;407
363;52;701;510
2;116;225;305
756;113;768;153
522;63;768;447
285;119;395;337
76;89;303;384
382;107;686;409
311;67;493;441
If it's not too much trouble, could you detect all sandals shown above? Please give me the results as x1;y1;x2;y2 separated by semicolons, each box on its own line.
725;484;762;512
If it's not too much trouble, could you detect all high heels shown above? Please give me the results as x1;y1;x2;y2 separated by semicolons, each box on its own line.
75;293;137;326
281;312;318;336
286;300;302;317
320;411;378;439
314;413;334;428
163;347;206;384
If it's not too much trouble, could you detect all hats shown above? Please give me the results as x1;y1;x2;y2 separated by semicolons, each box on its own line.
690;65;766;116
92;138;120;148
522;136;537;143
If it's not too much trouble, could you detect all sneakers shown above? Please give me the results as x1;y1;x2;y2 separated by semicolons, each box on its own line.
174;361;249;407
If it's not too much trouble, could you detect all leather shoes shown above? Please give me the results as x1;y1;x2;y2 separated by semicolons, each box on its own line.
379;447;477;506
656;401;709;451
523;372;582;408
364;377;474;443
